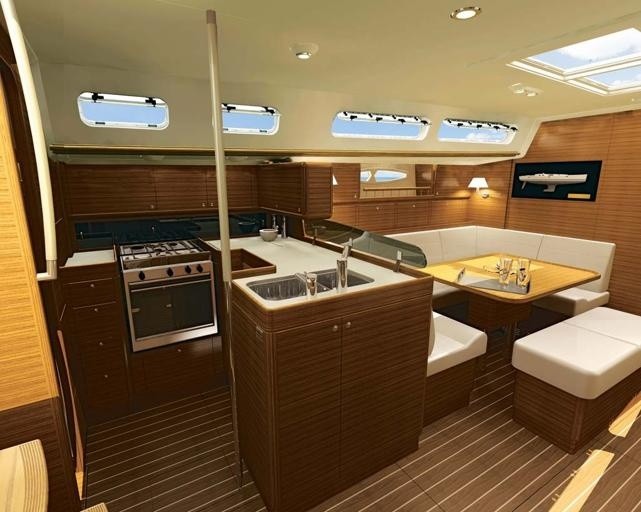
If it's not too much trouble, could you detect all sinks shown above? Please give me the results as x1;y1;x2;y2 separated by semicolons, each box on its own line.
246;274;326;302
315;268;374;289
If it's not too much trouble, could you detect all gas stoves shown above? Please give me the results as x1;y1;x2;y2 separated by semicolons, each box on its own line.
119;240;213;280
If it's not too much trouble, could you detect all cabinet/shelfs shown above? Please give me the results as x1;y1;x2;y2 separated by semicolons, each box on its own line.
233;293;434;512
335;162;470;235
64;165;158;221
63;262;130;429
258;162;333;219
154;166;257;219
132;336;229;409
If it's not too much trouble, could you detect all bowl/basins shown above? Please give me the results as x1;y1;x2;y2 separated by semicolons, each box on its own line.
259;229;279;241
238;221;255;232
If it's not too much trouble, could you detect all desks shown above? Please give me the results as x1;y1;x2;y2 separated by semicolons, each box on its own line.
414;251;600;353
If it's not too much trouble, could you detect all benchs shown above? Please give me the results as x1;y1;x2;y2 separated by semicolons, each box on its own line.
513;306;641;453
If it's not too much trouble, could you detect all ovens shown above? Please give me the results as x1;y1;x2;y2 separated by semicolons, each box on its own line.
124;275;219;352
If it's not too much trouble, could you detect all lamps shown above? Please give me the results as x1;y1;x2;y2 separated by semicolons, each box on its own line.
468;177;489;198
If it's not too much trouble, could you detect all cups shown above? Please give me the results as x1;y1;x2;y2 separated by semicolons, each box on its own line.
516;258;531;289
498;256;513;286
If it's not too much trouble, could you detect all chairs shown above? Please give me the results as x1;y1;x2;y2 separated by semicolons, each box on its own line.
425;310;487;425
475;226;617;332
386;227;476;315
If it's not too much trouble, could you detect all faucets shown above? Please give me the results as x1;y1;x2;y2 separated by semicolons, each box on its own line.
336;245;349;289
295;272;319;298
